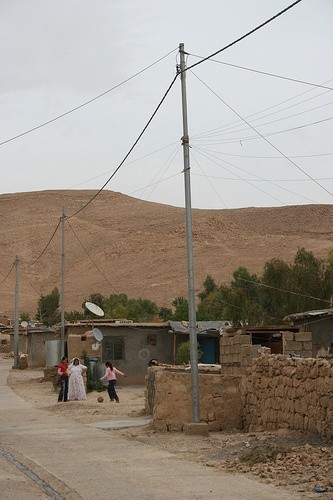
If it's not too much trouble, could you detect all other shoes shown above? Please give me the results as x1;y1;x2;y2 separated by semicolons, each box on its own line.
57;400;68;402
109;399;120;403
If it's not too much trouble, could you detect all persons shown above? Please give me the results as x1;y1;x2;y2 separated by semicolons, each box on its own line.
67;357;87;401
98;361;127;403
57;356;68;402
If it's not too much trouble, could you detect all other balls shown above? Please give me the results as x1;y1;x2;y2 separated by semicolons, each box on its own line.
98;397;103;402
